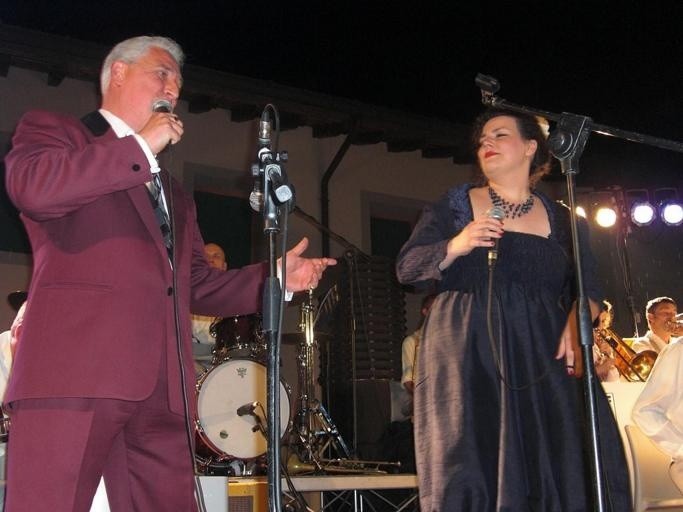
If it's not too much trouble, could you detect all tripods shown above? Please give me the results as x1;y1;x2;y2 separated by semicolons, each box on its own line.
279;305;364;473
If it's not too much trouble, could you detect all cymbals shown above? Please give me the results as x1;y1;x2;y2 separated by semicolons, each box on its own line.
8;291;28;311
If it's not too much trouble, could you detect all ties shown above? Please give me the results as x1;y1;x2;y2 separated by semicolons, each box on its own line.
149;173;173;262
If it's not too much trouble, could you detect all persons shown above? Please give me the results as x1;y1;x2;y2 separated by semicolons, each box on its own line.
400;293;439;474
593;297;683;463
0;299;28;419
189;243;228;344
396;110;631;512
2;34;338;512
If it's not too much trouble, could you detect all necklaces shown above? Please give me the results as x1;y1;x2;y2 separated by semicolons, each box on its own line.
488;187;535;219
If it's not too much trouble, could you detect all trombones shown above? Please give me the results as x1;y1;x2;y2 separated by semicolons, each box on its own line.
594;326;658;382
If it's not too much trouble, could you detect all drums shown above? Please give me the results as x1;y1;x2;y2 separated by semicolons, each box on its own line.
209;313;265;359
194;359;291;462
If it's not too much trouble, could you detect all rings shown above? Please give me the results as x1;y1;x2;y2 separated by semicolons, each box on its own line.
309;283;315;290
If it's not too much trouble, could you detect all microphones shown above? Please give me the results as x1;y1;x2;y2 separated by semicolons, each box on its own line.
237;402;260;416
152;99;173;151
487;206;506;267
249;111;277;212
